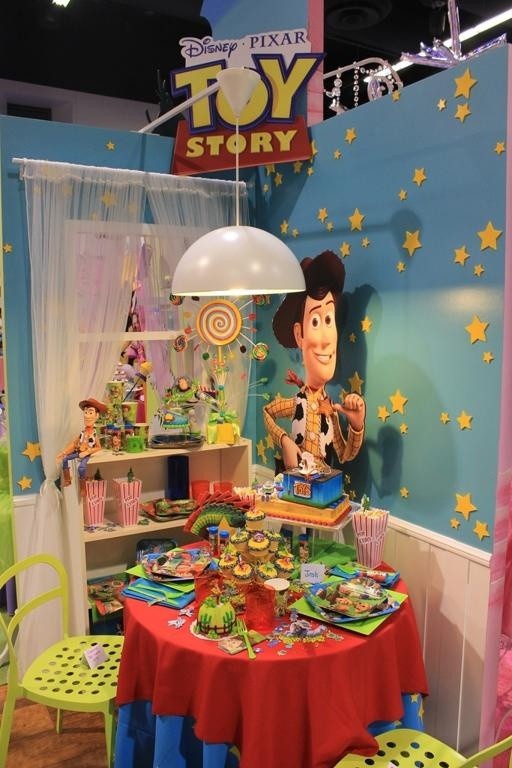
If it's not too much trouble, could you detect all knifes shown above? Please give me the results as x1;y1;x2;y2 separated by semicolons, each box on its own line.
128;586;179;606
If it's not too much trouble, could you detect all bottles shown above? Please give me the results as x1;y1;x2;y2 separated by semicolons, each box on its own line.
297;533;310;563
209;526;218;554
219;530;230;556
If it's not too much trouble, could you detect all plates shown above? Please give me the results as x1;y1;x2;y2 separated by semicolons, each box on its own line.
151;546;213;580
154;498;198;517
301;575;401;625
138;548;219;583
138;496;191;522
310;579;389;621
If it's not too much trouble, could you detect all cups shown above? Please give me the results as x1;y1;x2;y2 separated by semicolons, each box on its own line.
213;481;233;496
191;479;210;506
263;577;290;619
107;380;125;403
126;436;146;453
121;401;138;425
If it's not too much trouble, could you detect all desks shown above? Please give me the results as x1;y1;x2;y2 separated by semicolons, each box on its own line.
112;532;429;768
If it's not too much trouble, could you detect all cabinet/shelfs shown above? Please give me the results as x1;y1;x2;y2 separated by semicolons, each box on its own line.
56;436;253;635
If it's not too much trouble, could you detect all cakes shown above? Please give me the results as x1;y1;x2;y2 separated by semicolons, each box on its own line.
193;511;293;639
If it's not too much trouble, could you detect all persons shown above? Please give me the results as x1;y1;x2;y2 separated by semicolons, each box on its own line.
58;398;108;496
166;376;218;424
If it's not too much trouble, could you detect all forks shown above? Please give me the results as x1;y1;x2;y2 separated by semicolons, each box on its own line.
134;583;184;598
234;616;257;659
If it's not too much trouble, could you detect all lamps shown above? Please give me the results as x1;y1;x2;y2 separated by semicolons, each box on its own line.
138;67;306;296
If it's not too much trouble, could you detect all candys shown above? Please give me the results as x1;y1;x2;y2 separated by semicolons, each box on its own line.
169;293;270;392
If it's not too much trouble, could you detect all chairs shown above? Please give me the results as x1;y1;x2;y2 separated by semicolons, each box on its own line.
334;728;512;767
0;553;124;767
